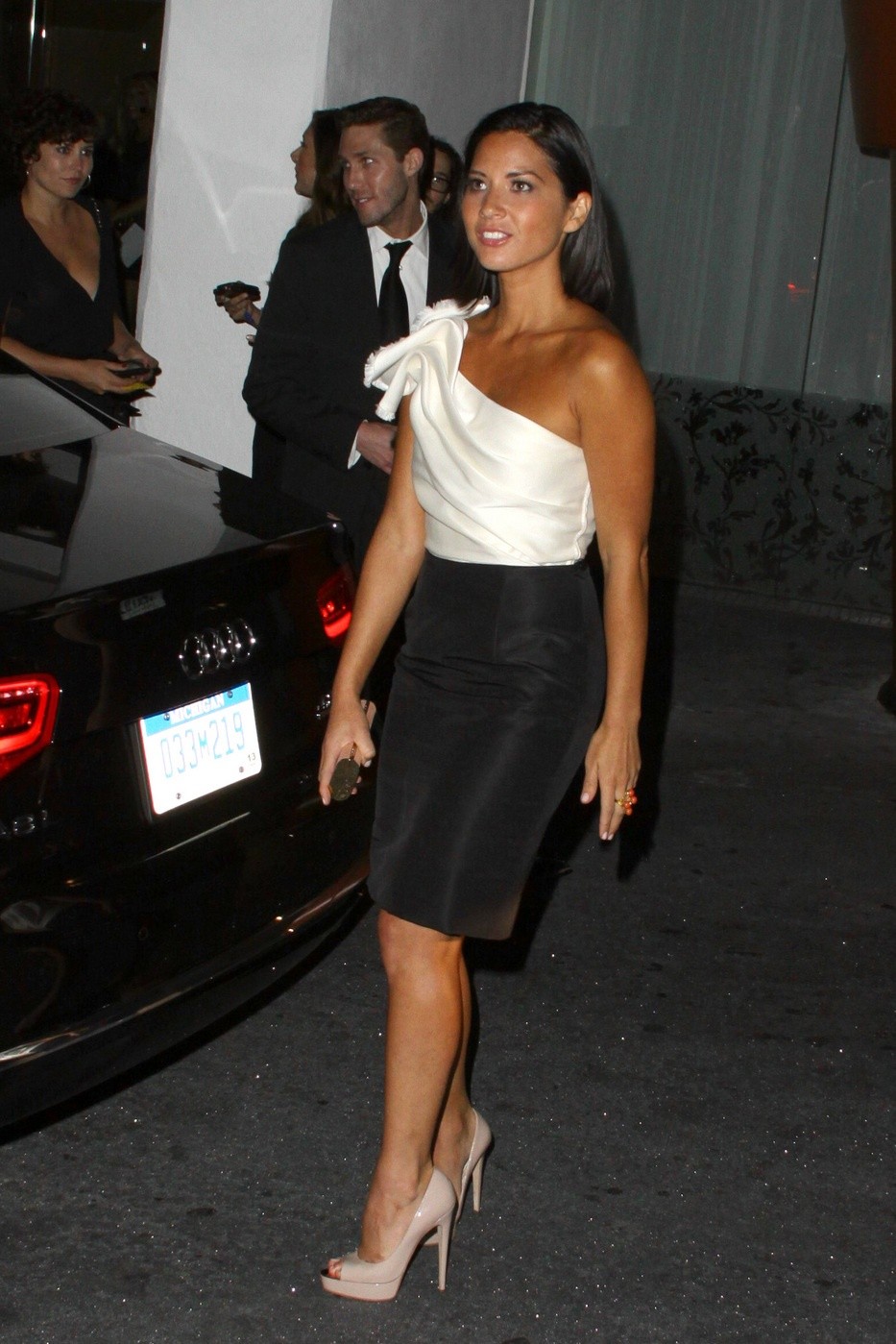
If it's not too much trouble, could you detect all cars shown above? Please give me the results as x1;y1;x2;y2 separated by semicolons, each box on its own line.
0;346;412;1135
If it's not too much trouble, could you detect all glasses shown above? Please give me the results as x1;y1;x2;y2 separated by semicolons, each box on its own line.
431;173;449;194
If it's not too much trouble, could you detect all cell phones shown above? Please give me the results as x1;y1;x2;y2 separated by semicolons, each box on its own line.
111;360;149;376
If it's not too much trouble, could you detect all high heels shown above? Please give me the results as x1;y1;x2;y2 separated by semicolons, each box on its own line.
318;1170;457;1301
423;1107;492;1244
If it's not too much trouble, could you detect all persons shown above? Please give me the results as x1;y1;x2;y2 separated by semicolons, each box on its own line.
274;96;492;711
426;139;462;208
0;109;159;426
212;109;338;477
320;103;652;1298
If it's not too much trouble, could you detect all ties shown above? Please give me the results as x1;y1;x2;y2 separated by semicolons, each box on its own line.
376;241;414;347
